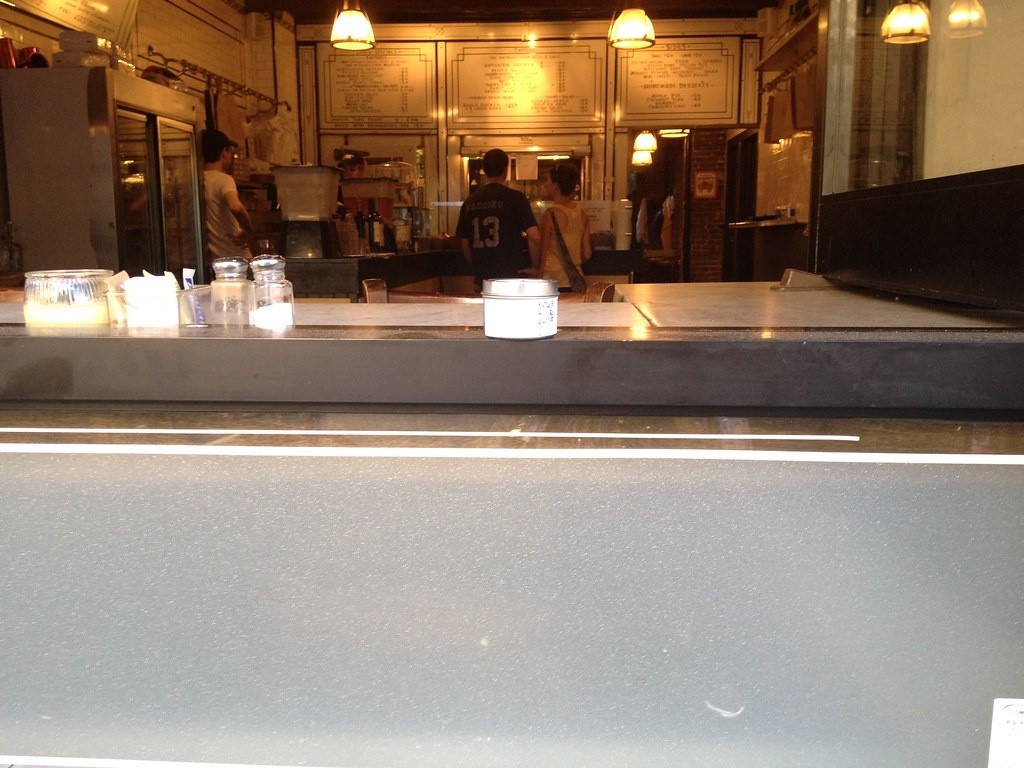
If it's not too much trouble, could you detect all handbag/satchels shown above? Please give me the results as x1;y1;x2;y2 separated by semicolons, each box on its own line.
548;208;587;293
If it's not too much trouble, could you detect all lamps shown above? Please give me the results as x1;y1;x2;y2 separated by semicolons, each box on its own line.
880;0;931;44
606;0;656;51
658;129;690;138
329;0;377;51
633;130;658;152
949;0;987;40
632;151;653;166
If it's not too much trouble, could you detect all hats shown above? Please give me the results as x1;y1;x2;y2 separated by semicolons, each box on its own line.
202;129;240;151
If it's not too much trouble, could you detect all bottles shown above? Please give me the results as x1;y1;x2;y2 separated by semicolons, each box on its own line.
210;254;256;329
352;197;386;253
249;254;296;331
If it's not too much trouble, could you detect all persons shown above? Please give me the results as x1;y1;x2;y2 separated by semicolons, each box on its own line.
455;148;593;293
202;129;255;280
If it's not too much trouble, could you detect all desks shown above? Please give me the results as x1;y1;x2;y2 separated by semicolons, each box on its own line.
728;221;807;283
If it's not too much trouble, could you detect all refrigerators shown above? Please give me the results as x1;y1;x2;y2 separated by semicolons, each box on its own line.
0;67;210;286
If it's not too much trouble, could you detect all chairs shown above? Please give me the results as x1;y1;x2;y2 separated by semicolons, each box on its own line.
361;278;388;304
582;280;615;303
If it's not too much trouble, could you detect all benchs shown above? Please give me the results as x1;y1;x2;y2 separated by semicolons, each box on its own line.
389;289;583;304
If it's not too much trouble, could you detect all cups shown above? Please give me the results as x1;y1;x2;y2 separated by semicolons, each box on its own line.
179;284;212;329
108;291;129;329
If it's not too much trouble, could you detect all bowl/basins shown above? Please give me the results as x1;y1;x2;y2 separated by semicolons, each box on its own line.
22;270;114;332
482;278;559;339
